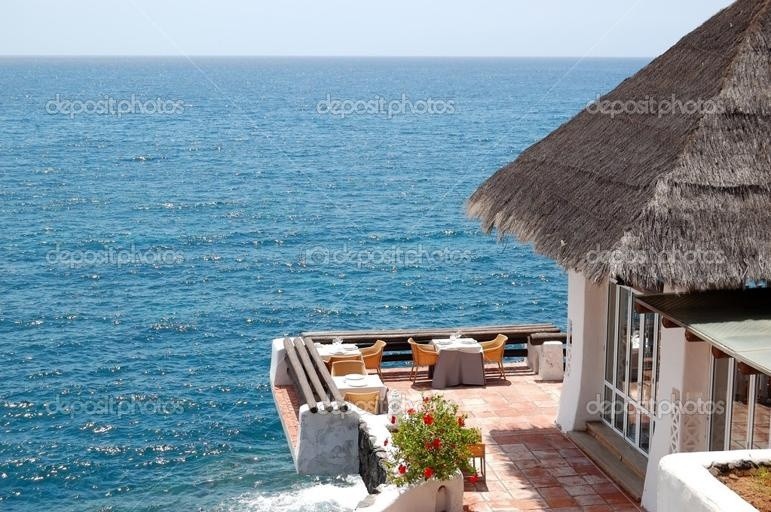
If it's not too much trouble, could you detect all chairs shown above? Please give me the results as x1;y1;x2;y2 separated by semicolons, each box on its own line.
314;333;510;414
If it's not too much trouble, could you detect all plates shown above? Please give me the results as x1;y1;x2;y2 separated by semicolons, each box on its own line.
346;373;368;387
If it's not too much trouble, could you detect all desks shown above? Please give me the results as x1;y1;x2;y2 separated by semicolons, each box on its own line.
464;429;487;480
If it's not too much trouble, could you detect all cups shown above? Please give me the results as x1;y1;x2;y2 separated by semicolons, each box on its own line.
450;329;462;344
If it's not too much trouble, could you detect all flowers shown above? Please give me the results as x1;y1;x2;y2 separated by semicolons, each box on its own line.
380;394;481;482
